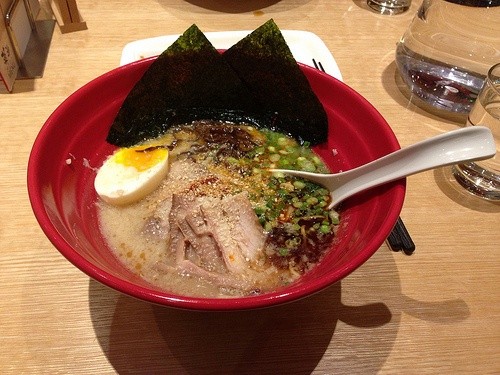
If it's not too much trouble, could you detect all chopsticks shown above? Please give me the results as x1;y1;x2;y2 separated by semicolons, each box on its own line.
312;59;416;257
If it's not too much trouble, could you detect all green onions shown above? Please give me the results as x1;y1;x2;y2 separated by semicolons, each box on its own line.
226;124;341;251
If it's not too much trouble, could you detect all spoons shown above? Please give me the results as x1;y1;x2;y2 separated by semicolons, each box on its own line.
266;126;496;211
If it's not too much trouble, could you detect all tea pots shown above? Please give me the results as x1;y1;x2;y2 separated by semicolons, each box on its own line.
393;0;500;115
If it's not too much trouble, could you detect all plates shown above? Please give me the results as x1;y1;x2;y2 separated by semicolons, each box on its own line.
119;29;343;81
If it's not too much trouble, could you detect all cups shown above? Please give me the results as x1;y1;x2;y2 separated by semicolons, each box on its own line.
366;0;411;16
452;62;500;203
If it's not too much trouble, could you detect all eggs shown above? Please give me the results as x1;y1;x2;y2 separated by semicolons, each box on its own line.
93;145;169;205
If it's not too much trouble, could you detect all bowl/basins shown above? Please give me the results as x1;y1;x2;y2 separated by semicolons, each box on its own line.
26;49;405;313
